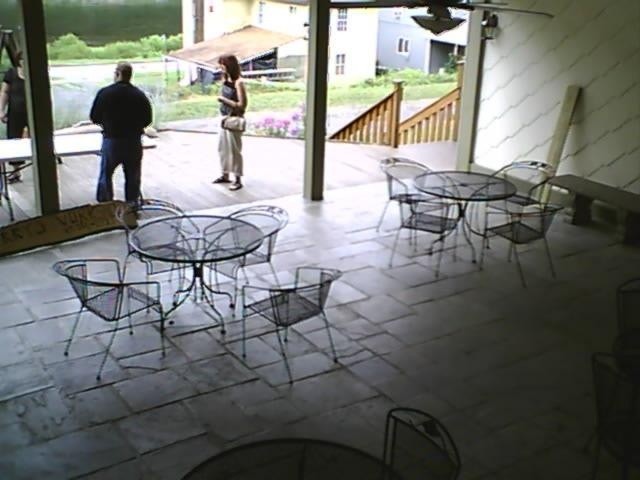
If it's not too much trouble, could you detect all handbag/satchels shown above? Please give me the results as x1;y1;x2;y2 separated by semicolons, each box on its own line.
220;116;246;132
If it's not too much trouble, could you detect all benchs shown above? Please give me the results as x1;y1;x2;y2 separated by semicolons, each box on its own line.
547;174;640;234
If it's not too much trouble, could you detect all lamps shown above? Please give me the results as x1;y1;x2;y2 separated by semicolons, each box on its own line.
481;14;498;39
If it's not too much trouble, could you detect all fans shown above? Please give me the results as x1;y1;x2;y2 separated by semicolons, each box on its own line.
326;0;553;36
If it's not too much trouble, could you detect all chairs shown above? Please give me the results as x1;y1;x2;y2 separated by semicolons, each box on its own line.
203;205;289;318
388;194;477;278
480;160;555;250
376;156;445;246
482;203;564;289
614;278;640;378
242;267;343;385
381;405;461;480
50;257;165;383
114;199;200;303
584;351;640;480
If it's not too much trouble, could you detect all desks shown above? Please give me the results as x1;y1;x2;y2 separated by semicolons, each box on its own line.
128;215;263;335
183;436;397;480
0;130;157;222
411;171;516;264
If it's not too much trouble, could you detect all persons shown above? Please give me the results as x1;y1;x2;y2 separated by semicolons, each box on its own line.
89;61;152;204
0;49;30;183
212;55;247;191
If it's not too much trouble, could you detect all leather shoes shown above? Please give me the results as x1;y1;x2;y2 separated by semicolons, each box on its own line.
9;172;22;182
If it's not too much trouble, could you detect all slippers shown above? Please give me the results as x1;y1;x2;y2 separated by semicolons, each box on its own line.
229;182;243;190
212;175;232;183
